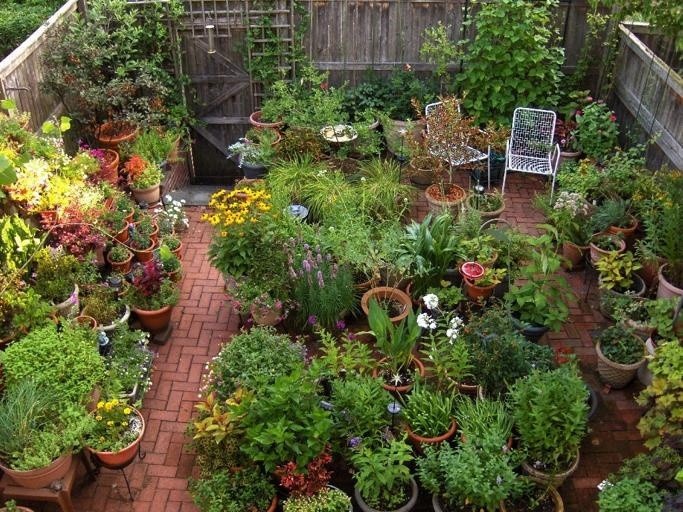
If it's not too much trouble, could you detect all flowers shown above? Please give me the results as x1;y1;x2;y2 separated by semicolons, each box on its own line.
552;190;593;245
553;117;576;150
81;399;146;467
226;136;266;169
185;183;536;512
569;95;621;162
101;315;161;408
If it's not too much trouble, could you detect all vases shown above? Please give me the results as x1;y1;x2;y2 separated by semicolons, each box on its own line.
239;159;270;179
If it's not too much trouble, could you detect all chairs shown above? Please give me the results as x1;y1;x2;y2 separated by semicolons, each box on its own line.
501;107;563;206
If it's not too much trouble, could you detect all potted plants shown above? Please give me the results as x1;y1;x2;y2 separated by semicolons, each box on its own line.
249;60;506;290
0;99;191;345
44;0;206;166
464;205;593;512
597;152;683;512
0;375;97;490
0;326;109;412
0;498;34;512
